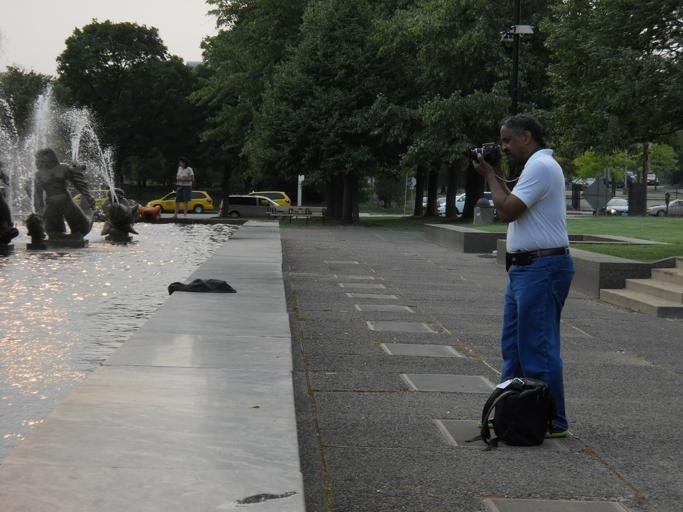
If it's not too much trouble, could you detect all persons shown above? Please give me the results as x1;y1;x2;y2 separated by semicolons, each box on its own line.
33;149;97;242
467;111;573;440
171;156;195;219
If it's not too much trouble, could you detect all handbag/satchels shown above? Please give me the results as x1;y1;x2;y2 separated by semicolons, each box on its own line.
479;375;552;449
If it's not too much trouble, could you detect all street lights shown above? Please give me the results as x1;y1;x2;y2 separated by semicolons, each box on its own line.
499;0;536;115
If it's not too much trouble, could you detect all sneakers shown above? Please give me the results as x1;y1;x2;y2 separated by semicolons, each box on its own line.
545;425;568;439
477;418;494;428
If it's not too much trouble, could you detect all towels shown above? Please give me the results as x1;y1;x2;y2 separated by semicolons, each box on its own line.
168;278;236;295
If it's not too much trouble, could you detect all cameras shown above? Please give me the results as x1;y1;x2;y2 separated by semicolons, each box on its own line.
471;141;501;165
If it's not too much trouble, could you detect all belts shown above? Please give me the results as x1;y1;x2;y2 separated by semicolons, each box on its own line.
502;247;569;263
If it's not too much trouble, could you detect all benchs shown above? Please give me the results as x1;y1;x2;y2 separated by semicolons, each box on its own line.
265;206;327;224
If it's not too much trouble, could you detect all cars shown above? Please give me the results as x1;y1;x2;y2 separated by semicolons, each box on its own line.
573;177;595;190
423;192;497;216
603;199;683;216
72;190;291;217
623;172;660;185
